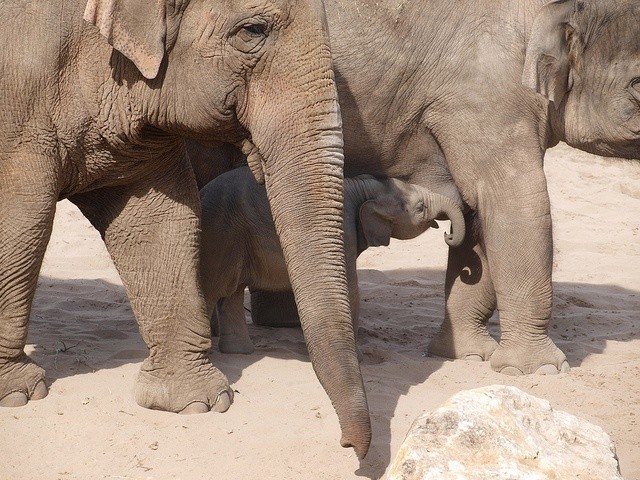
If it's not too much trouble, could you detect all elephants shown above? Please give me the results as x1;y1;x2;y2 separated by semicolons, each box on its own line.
199;164;466;355
186;0;639;381
0;0;375;464
